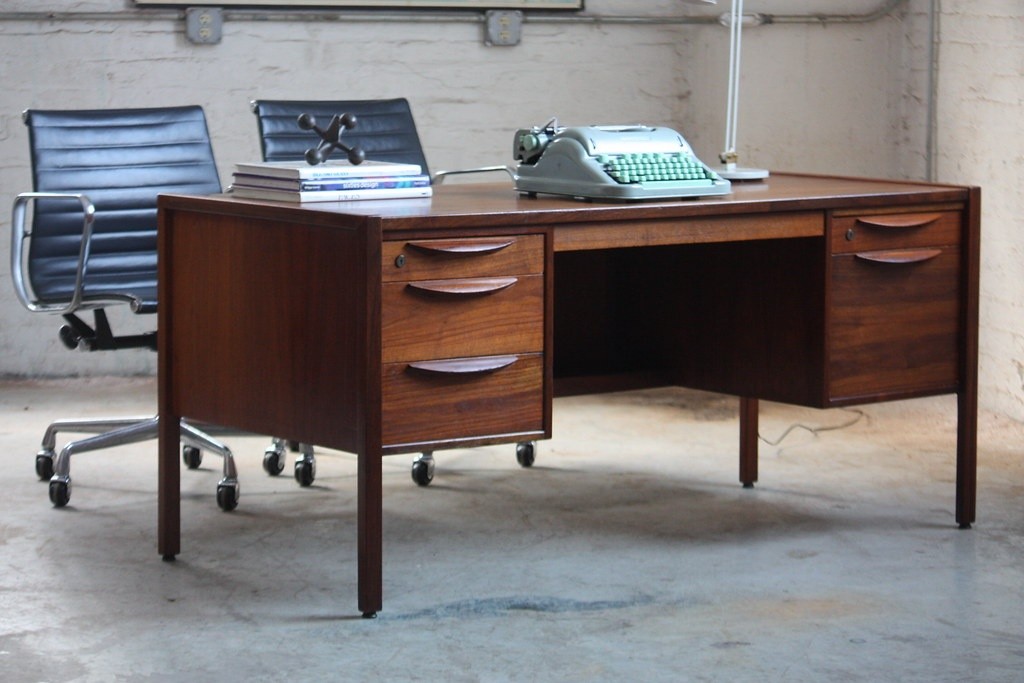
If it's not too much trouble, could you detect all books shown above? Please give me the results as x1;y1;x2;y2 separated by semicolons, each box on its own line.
230;159;433;202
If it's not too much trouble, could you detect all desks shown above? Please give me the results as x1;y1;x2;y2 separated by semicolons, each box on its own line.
157;171;982;620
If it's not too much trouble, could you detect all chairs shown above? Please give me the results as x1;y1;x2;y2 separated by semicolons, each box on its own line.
10;106;317;511
251;98;535;486
